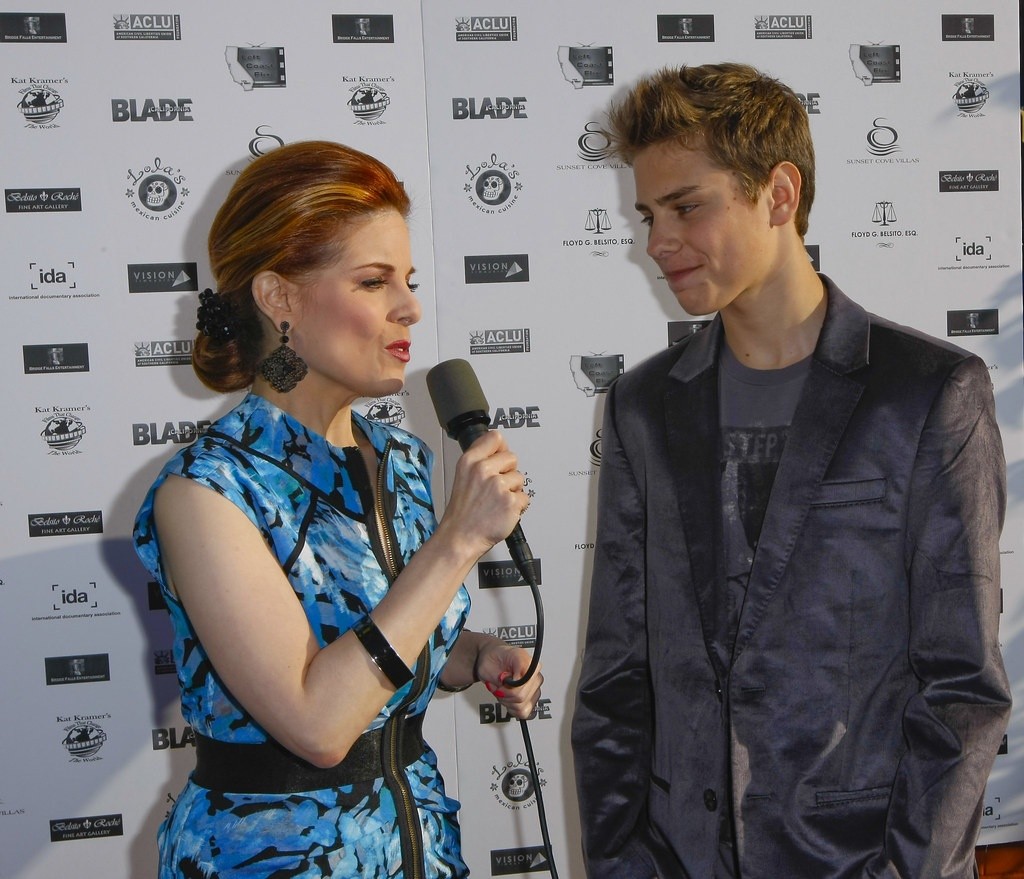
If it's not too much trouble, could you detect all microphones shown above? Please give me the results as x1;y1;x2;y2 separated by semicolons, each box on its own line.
427;359;534;583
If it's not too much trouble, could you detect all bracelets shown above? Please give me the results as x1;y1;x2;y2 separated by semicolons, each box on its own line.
350;615;415;691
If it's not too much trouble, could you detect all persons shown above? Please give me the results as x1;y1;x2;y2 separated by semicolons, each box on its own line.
132;141;543;879
569;62;1012;879
